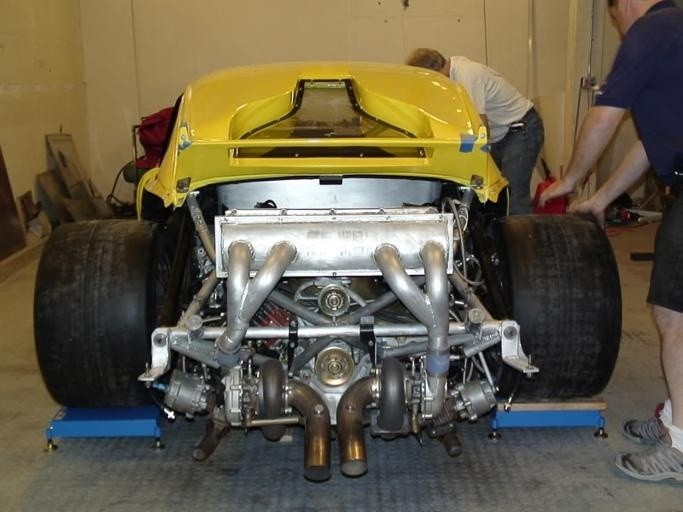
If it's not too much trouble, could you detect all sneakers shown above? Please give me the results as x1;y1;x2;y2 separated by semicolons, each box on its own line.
615;403;683;481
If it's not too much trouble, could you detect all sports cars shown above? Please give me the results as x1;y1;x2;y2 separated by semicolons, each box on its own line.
33;61;621;482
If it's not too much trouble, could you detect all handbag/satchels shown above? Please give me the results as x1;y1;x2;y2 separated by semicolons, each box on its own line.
510;121;526;133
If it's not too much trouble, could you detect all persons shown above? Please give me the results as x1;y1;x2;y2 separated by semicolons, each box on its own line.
408;49;545;217
535;0;683;483
